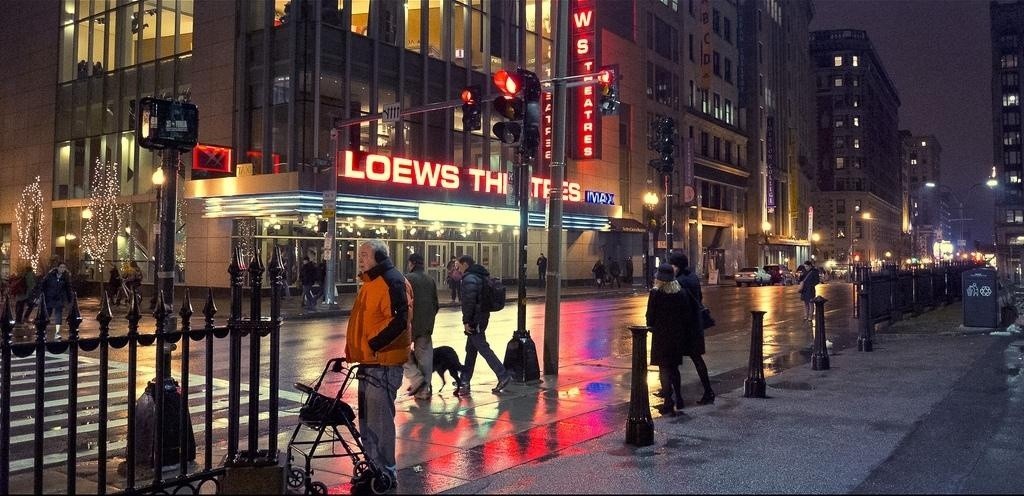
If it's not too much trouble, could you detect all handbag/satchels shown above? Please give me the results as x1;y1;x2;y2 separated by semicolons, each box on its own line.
700;304;716;329
798;280;804;292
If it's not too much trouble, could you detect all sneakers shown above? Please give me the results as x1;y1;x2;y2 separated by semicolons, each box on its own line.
407;375;512;400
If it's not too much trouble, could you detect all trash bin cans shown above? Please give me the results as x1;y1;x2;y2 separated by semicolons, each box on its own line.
963;266;999;328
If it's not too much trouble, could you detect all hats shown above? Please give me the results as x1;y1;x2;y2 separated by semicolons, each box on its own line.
654;263;674;282
671;253;688;267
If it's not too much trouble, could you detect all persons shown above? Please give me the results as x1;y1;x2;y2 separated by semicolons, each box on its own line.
653;252;715;404
592;258;634;289
344;239;415;483
798;261;819;321
281;256;510;401
15;260;143;339
537;254;547;287
646;262;690;410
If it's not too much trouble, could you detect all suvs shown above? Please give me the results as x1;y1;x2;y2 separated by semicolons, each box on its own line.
762;264;794;286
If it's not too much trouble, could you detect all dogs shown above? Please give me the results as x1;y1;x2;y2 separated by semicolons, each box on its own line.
407;346;475;396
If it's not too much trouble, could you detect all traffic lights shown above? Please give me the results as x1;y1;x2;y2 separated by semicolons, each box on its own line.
492;67;543;149
459;83;482;131
597;67;617;116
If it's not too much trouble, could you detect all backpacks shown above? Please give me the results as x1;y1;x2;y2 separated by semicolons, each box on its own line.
473;268;506;312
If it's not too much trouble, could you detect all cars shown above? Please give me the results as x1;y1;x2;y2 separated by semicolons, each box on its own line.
734;267;771;286
795;259;934;281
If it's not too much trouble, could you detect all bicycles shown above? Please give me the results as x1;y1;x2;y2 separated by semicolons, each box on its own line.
111;277;142;307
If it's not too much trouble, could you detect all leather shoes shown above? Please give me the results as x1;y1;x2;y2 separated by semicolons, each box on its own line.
652;385;715;414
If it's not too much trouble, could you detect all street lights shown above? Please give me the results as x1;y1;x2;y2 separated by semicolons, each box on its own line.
151;166;164;311
923;177;998;264
849;210;870;269
809;231;820;268
643;191;658;295
760;222;773;266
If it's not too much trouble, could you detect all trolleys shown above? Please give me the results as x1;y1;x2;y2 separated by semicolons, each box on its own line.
285;355;400;494
